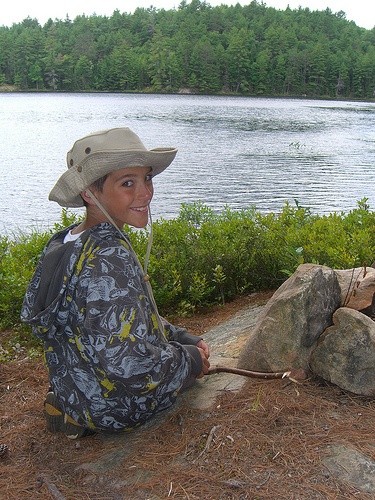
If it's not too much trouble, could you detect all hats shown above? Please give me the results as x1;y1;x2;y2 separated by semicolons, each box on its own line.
49;127;177;207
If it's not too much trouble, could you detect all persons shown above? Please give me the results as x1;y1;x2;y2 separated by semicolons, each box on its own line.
24;127;210;438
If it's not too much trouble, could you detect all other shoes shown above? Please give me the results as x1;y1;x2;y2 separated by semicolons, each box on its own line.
64;412;96;440
43;393;63;433
